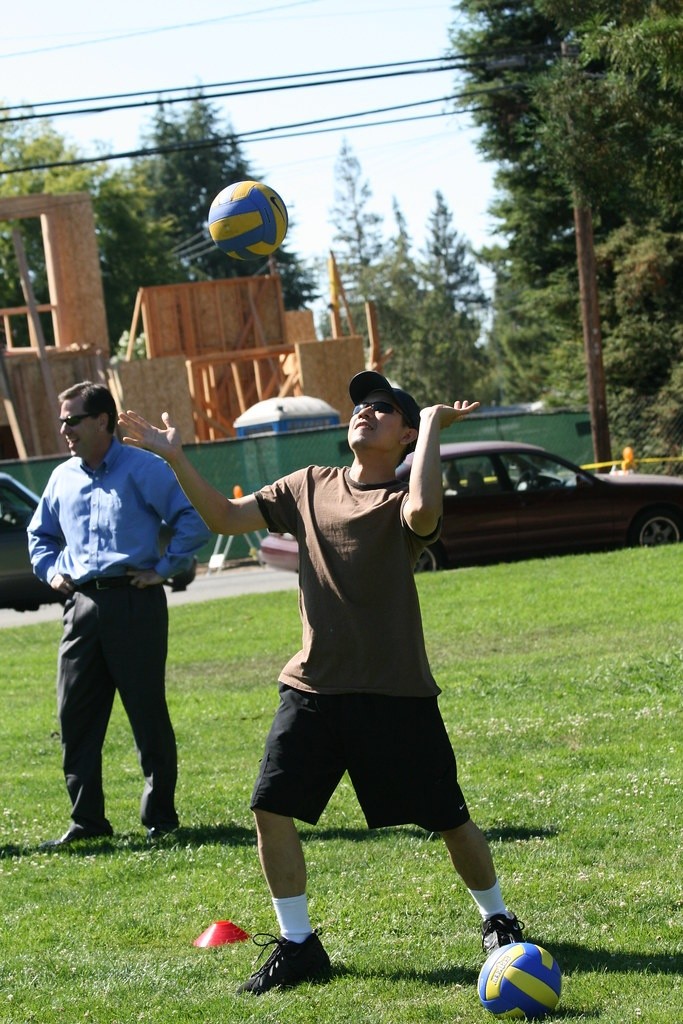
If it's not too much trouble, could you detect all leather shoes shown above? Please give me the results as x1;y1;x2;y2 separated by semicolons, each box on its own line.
147;823;178;847
38;829;78;849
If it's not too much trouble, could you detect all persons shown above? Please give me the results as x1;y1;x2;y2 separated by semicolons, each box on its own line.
117;369;528;997
25;381;213;847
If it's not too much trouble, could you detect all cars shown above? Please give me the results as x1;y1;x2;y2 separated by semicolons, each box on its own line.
0;472;69;612
259;440;683;574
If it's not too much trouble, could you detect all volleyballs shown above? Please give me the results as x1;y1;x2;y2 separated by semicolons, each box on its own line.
206;179;290;264
475;941;563;1021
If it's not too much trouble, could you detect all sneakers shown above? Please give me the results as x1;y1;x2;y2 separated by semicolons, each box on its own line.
237;925;330;997
480;910;524;956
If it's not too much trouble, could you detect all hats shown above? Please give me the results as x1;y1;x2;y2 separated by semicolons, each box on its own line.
349;370;420;456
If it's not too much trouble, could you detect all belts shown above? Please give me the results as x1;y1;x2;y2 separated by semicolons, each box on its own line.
81;575;129;591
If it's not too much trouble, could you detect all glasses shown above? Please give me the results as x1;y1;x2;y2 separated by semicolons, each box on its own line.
352;403;412;427
59;413;100;427
